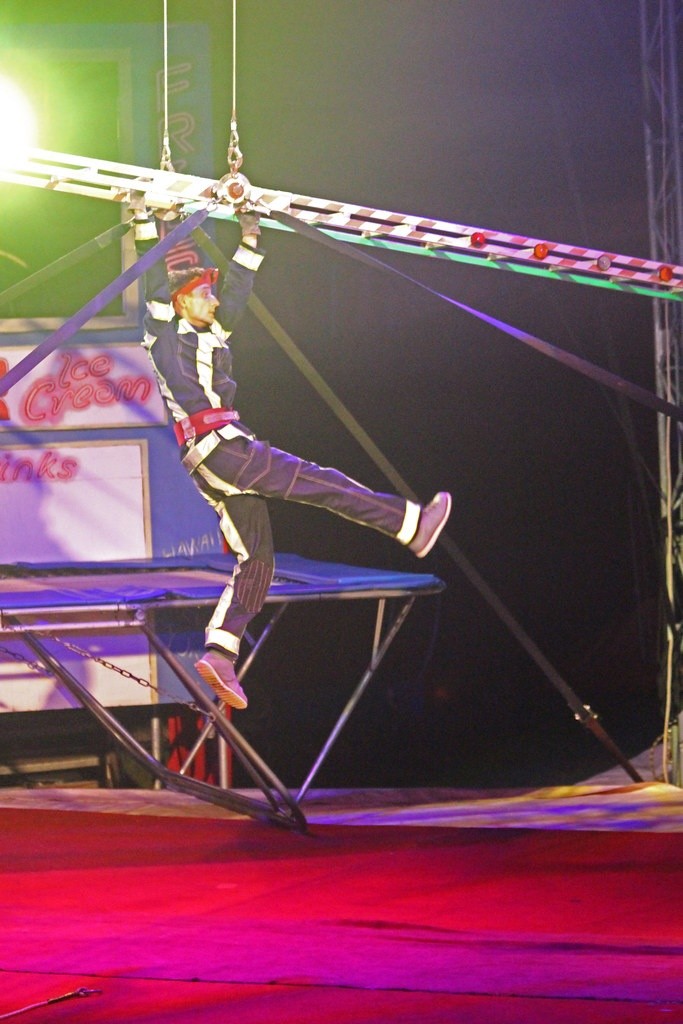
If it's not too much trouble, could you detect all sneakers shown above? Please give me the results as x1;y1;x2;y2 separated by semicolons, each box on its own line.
407;492;452;558
194;649;247;709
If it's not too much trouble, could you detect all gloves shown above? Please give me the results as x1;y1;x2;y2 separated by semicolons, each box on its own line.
128;177;152;213
235;199;263;236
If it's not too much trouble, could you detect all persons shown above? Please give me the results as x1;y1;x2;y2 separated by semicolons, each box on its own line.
127;176;453;710
